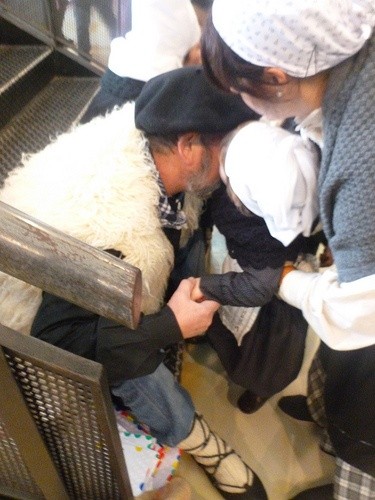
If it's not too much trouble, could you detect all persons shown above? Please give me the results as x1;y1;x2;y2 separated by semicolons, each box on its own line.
0;65;268;500
48;0;74;46
171;120;320;414
78;0;201;125
201;0;375;500
74;0;119;57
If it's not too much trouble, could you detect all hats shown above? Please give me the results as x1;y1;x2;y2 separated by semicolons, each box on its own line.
210;0;374;81
223;114;326;248
134;65;262;139
131;0;203;66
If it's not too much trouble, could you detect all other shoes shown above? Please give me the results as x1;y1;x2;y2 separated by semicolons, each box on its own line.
278;395;313;422
237;389;274;414
187;337;203;343
292;484;336;500
179;411;267;500
